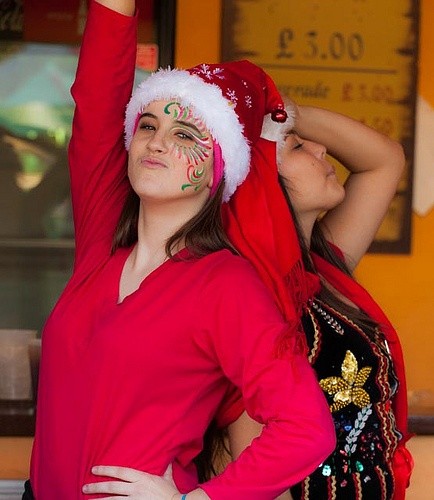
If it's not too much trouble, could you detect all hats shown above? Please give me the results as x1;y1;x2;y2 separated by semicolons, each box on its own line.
126;59;296;204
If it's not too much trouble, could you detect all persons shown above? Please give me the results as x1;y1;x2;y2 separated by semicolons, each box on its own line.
20;0;338;500
197;91;420;500
